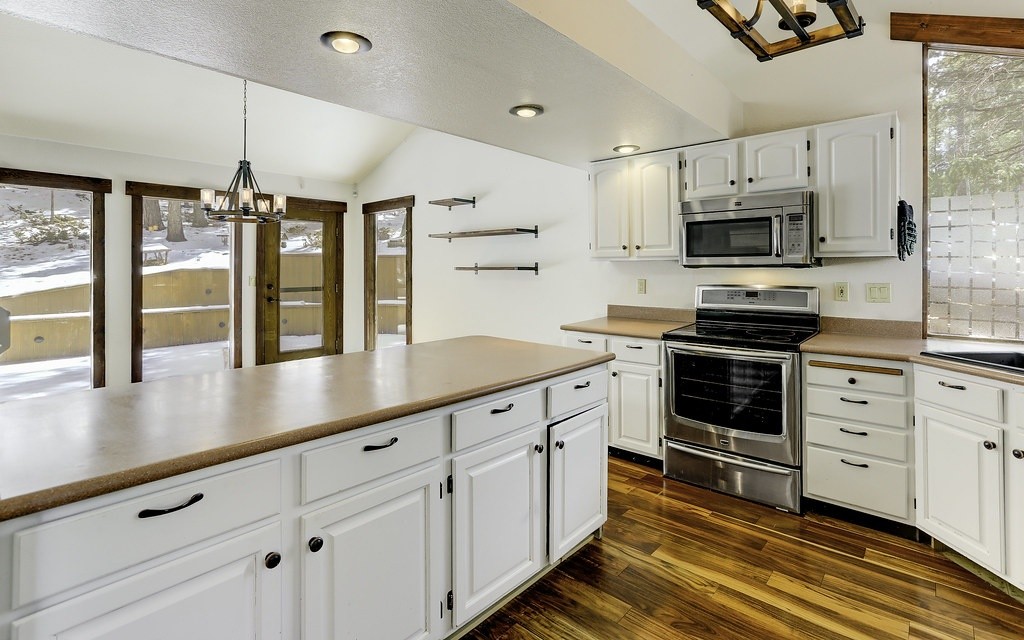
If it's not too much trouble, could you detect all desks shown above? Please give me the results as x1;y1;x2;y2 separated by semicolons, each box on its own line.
142;242;171;266
217;233;227;246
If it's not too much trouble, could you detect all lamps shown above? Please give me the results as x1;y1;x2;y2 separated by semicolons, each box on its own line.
697;0;866;63
199;78;286;223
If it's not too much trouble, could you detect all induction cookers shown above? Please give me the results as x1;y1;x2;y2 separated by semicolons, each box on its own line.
660;284;822;353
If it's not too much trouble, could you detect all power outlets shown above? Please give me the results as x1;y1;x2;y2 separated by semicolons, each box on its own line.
834;282;849;301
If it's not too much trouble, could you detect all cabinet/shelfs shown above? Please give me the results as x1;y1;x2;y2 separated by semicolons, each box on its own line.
1;408;442;640
812;110;898;257
802;352;918;540
680;127;811;202
587;147;679;261
562;331;664;469
429;196;539;276
912;363;1024;603
439;364;611;640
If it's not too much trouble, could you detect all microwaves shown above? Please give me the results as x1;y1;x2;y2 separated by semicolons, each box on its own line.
678;191;823;268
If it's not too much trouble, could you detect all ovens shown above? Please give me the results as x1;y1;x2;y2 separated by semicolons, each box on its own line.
660;341;808;517
